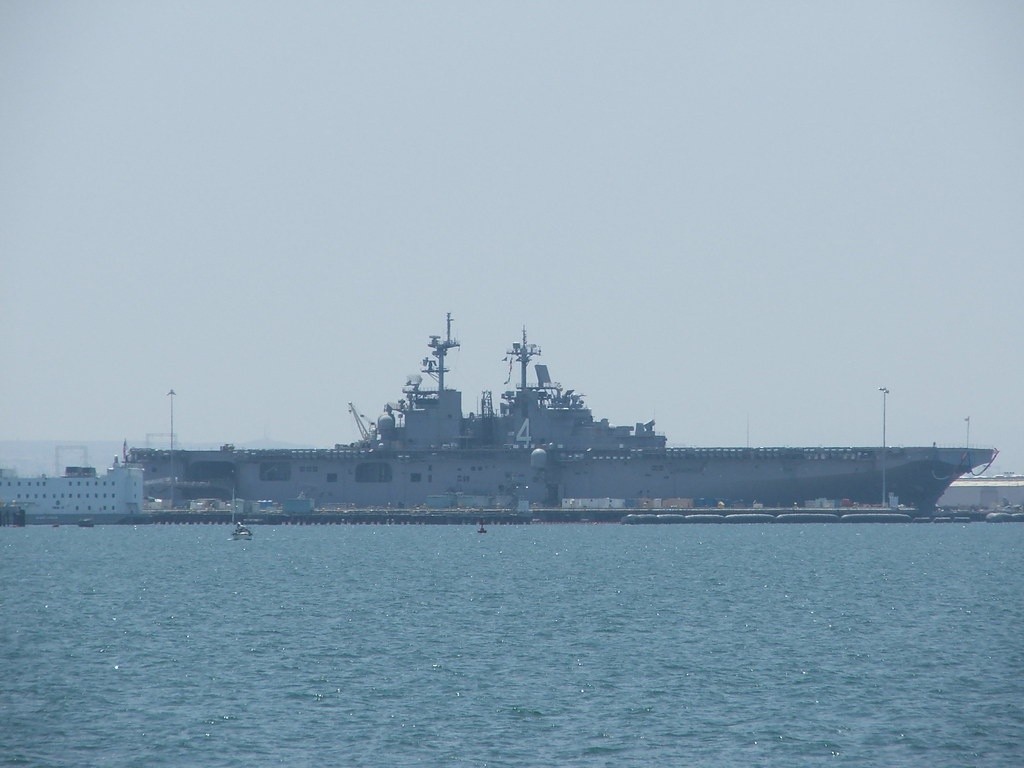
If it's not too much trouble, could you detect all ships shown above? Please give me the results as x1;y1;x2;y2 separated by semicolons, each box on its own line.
104;310;1000;512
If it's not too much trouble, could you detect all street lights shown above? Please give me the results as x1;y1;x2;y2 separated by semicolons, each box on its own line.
878;385;891;507
168;389;180;484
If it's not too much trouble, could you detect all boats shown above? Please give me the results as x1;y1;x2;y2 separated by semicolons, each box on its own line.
230;524;252;541
